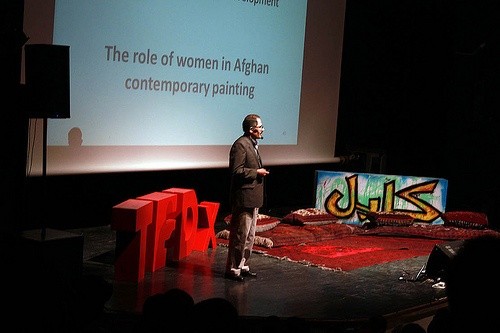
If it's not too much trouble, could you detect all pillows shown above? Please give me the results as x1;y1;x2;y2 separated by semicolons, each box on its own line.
223;213;281;232
441;211;488;228
367;211;415;226
282;209;339;225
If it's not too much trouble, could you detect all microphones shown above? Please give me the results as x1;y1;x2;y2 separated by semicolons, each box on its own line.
261;137;263;139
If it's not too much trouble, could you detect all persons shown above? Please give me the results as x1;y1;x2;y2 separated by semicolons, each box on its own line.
225;114;270;282
142;288;241;333
428;235;500;333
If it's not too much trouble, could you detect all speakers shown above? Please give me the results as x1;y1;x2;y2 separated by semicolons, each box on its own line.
426;240;464;279
24;44;70;119
